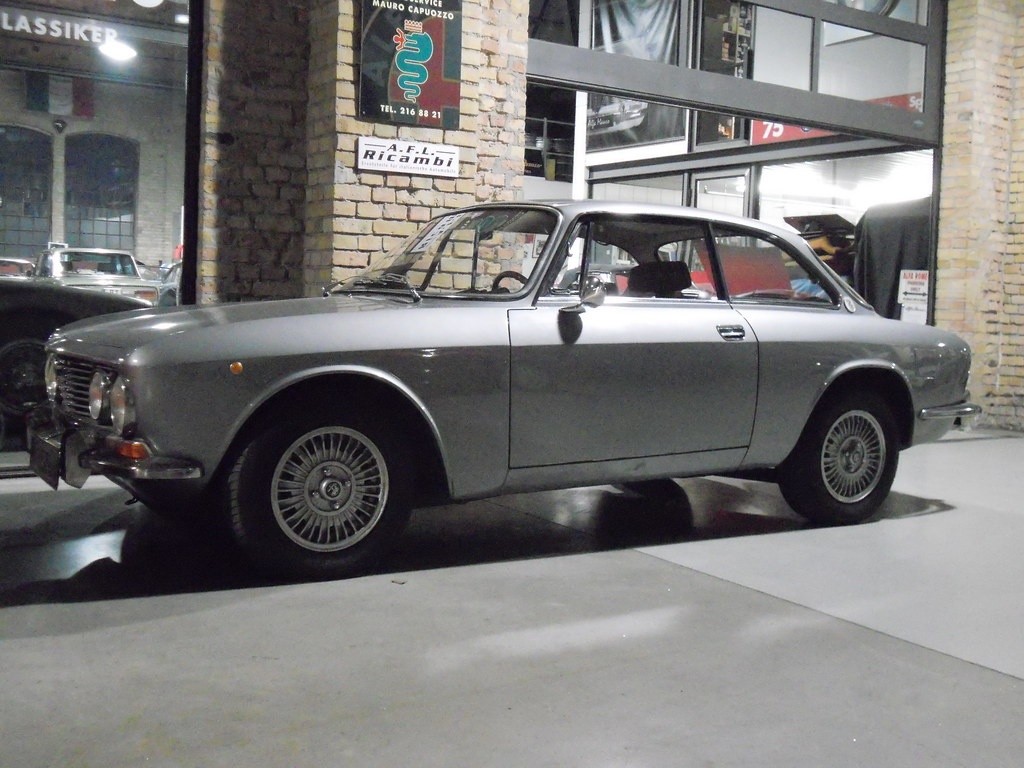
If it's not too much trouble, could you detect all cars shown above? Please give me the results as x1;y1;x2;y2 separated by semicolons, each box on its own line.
27;200;982;582
0;244;181;426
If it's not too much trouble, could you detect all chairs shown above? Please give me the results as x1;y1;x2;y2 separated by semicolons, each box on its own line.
97;262;118;274
627;261;692;298
61;260;73;272
